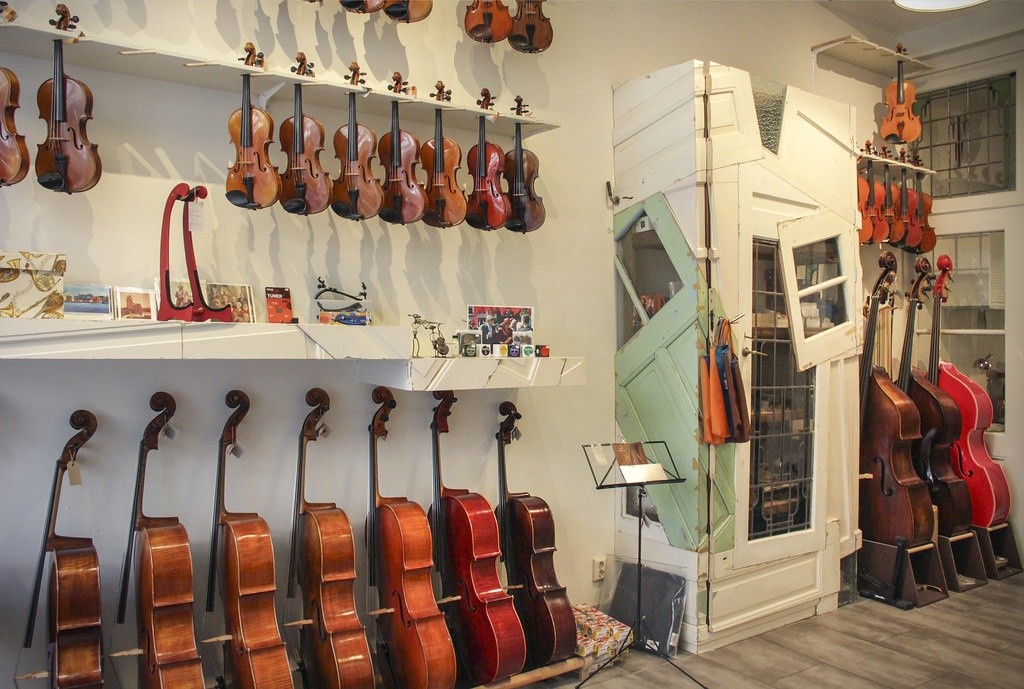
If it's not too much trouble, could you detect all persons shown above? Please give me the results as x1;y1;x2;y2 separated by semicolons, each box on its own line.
207;288;250;322
477;307;533;344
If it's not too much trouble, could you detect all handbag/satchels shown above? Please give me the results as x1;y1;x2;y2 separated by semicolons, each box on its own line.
700;318;751;446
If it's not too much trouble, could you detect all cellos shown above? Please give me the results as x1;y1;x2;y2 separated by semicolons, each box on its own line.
281;388;376;689
108;391;209;689
923;255;1011;529
426;390;528;685
495;401;579;672
200;388;296;689
12;409;105;689
893;256;972;537
363;385;457;689
859;251;935;549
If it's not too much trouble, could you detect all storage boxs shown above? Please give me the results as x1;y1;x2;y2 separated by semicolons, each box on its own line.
0;251;67;320
571;601;633;673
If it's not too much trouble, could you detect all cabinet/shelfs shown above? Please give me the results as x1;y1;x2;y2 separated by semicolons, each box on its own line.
814;35;935;175
0;23;584;391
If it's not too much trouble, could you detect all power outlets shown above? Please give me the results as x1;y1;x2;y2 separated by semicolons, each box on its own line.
593;559;606;582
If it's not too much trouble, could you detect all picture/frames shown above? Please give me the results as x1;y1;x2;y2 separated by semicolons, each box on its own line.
62;283;115;320
113;285;158;322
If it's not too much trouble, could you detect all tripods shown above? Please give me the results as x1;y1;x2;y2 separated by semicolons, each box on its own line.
574;441;708;689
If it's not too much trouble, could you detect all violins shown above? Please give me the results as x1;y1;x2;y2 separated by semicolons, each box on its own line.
35;3;103;194
278;52;334;217
420;80;468;229
225;42;283;211
338;0;554;54
466;87;546;232
378;71;429;226
857;43;938;254
0;1;31;189
331;62;385;221
